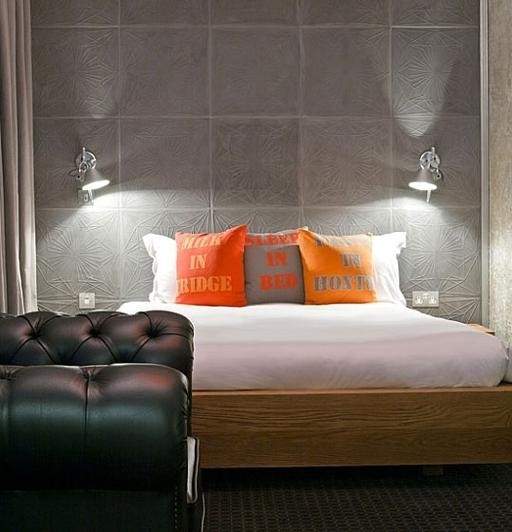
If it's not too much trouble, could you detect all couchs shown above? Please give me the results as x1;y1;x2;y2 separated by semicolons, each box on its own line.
1;310;208;532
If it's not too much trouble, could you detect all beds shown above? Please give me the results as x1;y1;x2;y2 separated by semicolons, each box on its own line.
115;301;512;468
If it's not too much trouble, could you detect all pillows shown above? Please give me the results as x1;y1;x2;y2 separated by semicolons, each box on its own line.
142;233;176;304
297;228;379;304
243;226;311;304
372;231;408;307
175;224;247;306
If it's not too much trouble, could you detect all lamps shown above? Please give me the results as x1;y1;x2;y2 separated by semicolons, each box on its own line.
407;146;442;203
68;147;110;206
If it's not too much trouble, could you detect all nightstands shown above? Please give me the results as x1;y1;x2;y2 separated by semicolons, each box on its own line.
467;323;495;335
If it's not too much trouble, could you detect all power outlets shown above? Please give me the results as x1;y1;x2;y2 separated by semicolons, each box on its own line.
412;291;440;308
